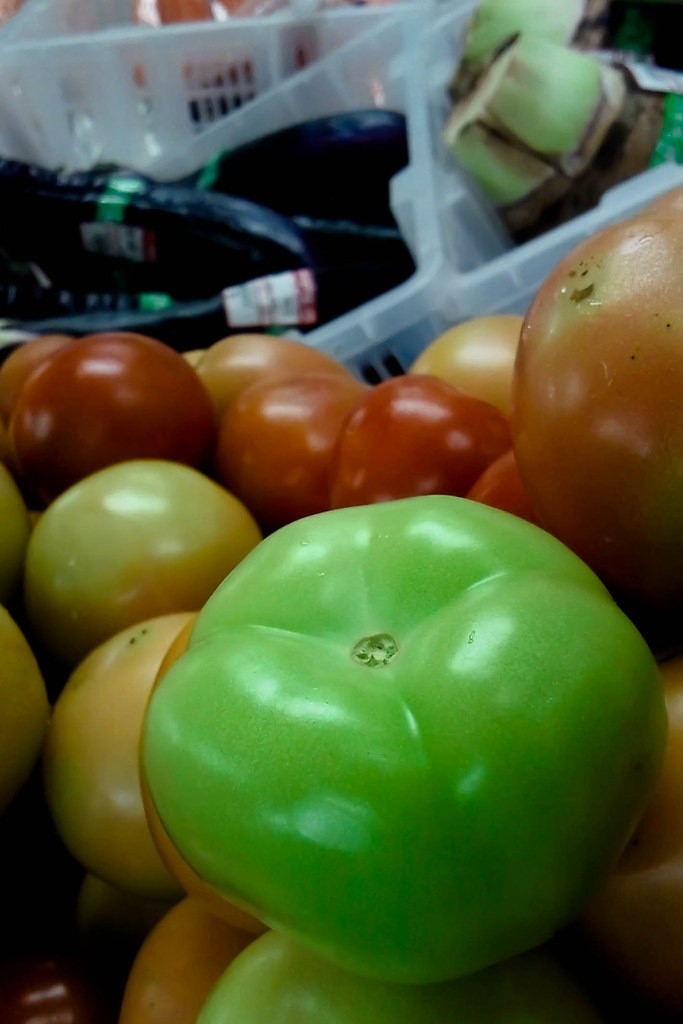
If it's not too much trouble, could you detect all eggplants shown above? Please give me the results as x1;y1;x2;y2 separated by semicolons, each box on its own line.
0;110;418;340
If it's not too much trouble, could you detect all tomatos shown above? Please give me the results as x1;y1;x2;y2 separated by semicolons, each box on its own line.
0;181;683;1024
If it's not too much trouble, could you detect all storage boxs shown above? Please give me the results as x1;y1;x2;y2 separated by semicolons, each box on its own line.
0;0;683;384
